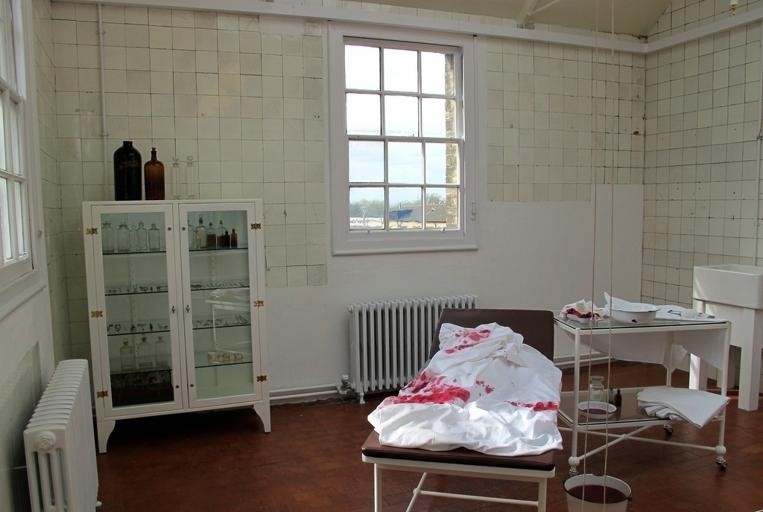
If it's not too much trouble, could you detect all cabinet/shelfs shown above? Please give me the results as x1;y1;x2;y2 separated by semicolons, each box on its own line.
81;199;270;454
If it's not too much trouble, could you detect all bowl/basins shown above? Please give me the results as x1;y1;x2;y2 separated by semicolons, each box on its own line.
607;302;660;325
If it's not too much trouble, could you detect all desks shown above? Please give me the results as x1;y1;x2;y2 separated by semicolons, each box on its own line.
552;304;731;475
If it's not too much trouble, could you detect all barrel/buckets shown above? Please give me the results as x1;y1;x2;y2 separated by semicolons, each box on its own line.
563;473;632;512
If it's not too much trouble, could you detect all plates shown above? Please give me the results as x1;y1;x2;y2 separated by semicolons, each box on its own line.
578;401;617;420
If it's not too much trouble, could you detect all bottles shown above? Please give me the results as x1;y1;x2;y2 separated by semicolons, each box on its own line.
100;216;238;253
120;336;169;371
113;139;166;200
588;375;622;409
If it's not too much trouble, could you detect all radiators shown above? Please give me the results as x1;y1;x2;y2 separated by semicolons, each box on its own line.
347;294;478;397
23;359;102;511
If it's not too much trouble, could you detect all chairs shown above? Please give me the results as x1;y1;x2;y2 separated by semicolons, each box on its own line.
362;308;555;512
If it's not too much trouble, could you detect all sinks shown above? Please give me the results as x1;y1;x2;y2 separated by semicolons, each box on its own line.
693;264;763;309
